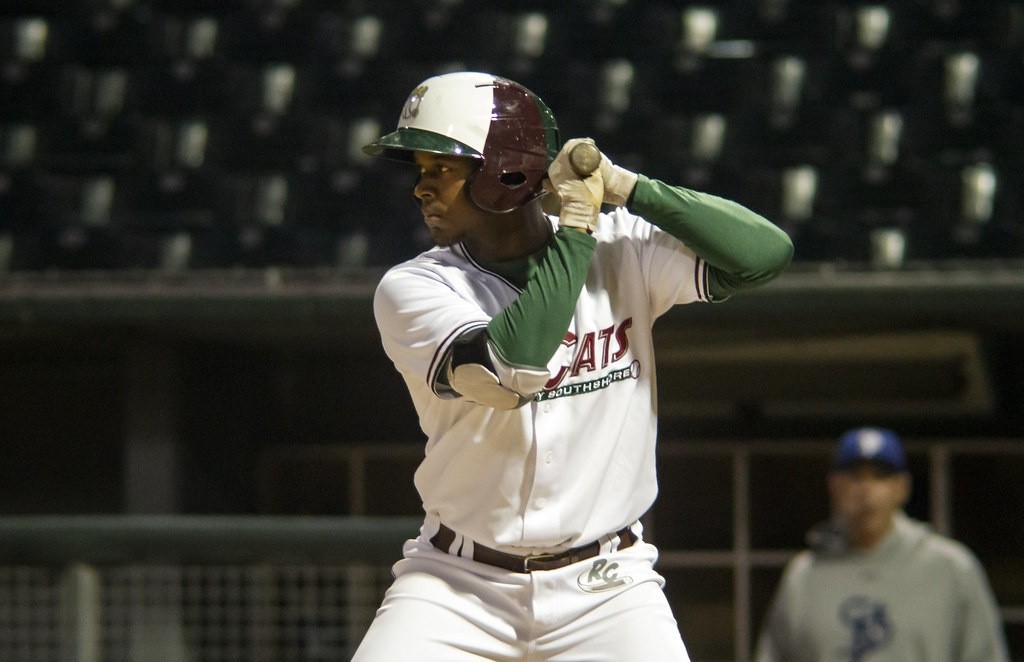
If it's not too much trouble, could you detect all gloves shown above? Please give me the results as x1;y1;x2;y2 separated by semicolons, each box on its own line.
548;137;639;233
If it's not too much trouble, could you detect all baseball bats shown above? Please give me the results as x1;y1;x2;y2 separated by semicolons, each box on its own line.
567;143;603;179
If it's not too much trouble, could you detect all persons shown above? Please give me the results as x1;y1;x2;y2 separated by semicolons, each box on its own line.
351;72;794;662
757;426;1007;662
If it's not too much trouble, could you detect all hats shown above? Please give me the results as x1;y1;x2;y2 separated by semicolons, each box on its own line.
836;429;903;473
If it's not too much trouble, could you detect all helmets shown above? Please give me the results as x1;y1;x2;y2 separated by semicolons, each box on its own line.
361;72;557;215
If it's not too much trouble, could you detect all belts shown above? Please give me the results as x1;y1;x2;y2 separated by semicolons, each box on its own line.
429;521;637;574
